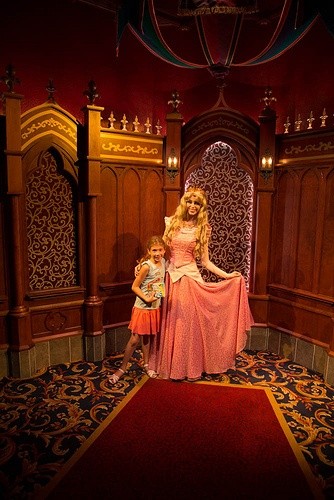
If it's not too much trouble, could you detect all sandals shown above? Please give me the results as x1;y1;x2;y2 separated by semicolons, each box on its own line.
109;369;125;385
143;364;157;378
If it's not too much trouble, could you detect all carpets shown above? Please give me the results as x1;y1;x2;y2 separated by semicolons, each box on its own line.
35;374;326;500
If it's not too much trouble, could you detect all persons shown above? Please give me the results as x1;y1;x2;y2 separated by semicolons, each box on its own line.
109;236;166;384
134;187;254;381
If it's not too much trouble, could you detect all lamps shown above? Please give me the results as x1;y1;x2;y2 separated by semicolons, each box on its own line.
166;147;180;184
260;156;273;185
